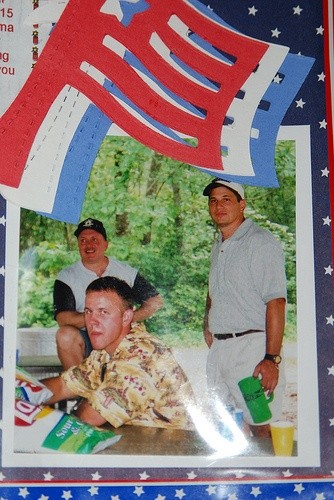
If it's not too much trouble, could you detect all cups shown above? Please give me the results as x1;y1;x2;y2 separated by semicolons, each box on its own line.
270;421;295;457
238;376;274;424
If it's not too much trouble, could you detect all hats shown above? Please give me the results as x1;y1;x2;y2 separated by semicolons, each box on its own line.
203;177;244;201
74;218;106;241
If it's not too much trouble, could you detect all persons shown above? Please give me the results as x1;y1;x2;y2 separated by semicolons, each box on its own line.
202;178;287;456
53;218;164;371
39;277;199;432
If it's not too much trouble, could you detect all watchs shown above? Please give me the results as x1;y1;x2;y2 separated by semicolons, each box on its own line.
264;353;281;364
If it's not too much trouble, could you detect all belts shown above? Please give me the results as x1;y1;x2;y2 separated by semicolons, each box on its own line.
214;329;263;340
